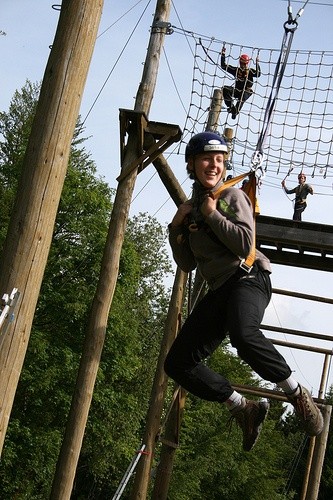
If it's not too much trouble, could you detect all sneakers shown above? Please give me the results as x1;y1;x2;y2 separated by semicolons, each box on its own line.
226;396;270;452
285;382;324;436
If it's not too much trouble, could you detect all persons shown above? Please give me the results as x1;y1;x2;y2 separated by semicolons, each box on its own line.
163;133;323;453
220;48;262;120
282;173;315;220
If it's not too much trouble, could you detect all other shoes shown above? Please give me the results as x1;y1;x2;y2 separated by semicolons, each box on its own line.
231;107;236;119
227;108;231;113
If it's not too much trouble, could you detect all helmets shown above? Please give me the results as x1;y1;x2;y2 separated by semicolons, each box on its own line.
185;132;227;162
240;55;249;64
298;174;305;178
